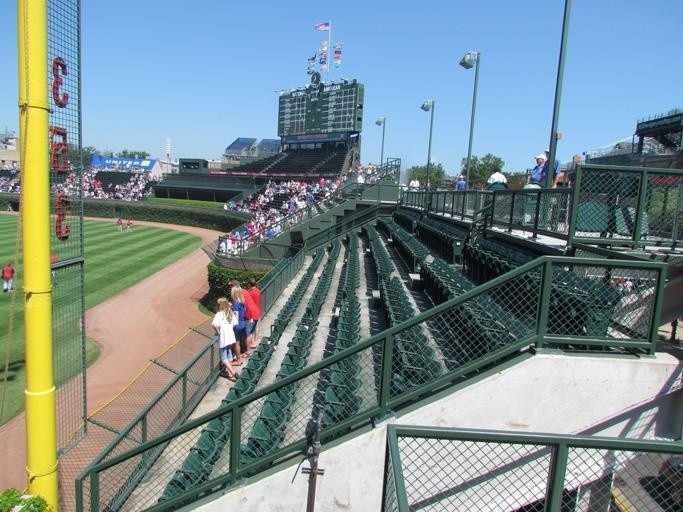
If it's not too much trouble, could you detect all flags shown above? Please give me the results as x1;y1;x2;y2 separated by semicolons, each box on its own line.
313;21;329;30
306;40;342;75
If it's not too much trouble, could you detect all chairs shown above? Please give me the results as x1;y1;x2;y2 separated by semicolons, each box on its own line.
158;201;648;511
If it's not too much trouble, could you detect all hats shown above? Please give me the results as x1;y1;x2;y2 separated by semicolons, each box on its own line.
228;280;240;287
248;278;255;284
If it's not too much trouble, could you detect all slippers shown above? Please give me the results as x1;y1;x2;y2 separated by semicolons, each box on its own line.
222;344;256;379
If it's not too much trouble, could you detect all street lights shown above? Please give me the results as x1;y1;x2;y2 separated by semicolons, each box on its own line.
420;99;435;212
459;51;481;215
375;115;387;179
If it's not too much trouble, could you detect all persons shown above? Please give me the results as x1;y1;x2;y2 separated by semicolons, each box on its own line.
611;275;634;295
50;251;58;279
486;168;507;185
126;218;132;233
216;160;380;257
528;149;561;228
210;277;262;380
0;163;21;193
454;175;469;191
117;218;122;233
409;176;433;192
1;262;14;292
49;167;161;202
